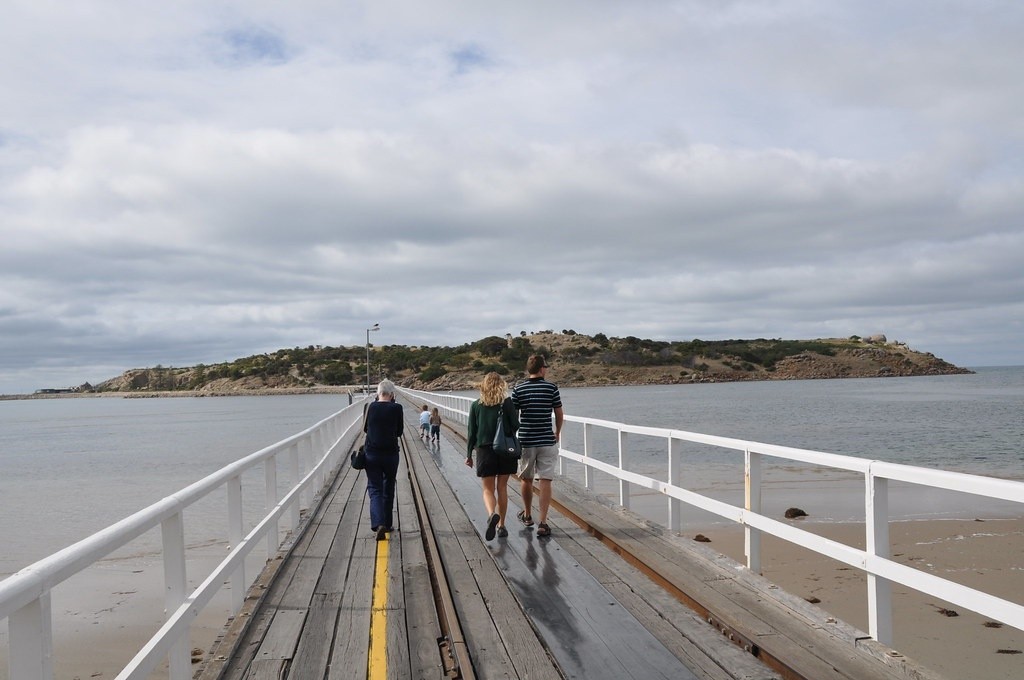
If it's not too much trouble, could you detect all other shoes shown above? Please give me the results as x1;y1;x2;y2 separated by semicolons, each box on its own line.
383;526;394;532
486;512;500;541
375;525;386;540
431;437;435;442
496;525;508;537
437;439;440;442
426;435;430;439
420;433;424;438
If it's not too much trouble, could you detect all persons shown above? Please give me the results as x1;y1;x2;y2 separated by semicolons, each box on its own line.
465;372;521;541
363;381;404;541
511;355;563;535
420;405;441;442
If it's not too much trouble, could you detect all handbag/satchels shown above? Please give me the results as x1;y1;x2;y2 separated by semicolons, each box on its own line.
493;404;521;459
351;446;367;469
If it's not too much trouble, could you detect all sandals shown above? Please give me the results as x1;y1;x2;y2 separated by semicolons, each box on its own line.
537;522;552;535
517;510;534;526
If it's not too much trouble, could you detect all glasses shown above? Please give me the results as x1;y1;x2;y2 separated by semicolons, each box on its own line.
541;365;550;370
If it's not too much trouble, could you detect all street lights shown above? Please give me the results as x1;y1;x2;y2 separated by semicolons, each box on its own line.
367;326;380;395
